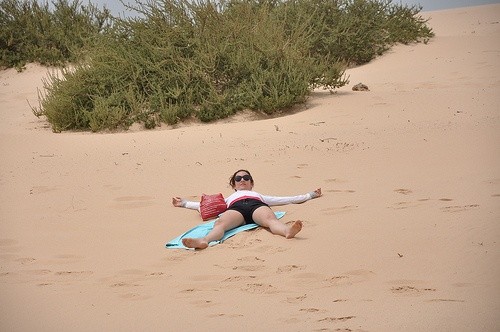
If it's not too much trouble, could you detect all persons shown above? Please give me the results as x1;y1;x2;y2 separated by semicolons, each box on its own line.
172;169;322;249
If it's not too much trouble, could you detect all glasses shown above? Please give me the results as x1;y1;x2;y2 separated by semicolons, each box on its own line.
233;175;252;184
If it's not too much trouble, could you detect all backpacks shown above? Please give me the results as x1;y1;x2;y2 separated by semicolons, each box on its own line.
200;193;227;221
